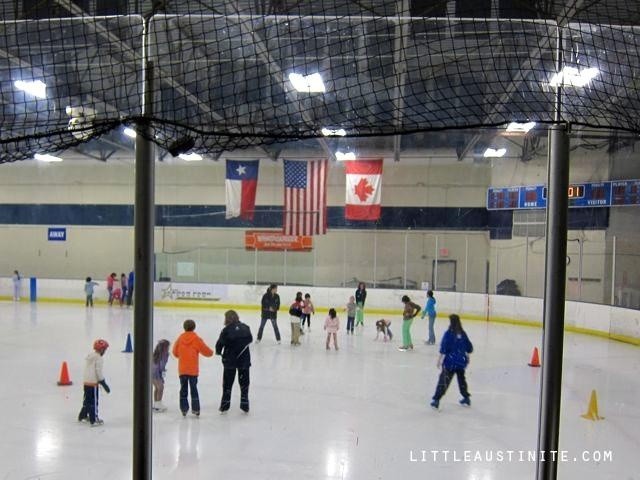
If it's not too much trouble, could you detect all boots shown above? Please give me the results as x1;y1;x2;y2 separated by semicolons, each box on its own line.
398;344;414;352
77;401;249;427
430;400;440;408
459;397;471;405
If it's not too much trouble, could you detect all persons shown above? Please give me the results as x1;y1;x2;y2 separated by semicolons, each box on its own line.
288;282;436;349
215;310;253;413
173;319;213;414
107;271;133;307
84;278;99;307
256;284;281;344
151;340;170;408
79;340;109;423
12;269;24;299
430;314;473;408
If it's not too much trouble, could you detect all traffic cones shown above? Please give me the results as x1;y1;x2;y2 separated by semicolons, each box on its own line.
581;386;608;421
56;360;75;386
528;348;541;368
121;334;134;353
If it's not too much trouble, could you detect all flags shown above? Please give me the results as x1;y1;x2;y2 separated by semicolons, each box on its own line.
283;159;328;236
224;159;258;221
345;160;383;220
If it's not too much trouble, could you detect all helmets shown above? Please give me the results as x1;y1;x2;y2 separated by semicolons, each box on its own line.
93;339;109;350
